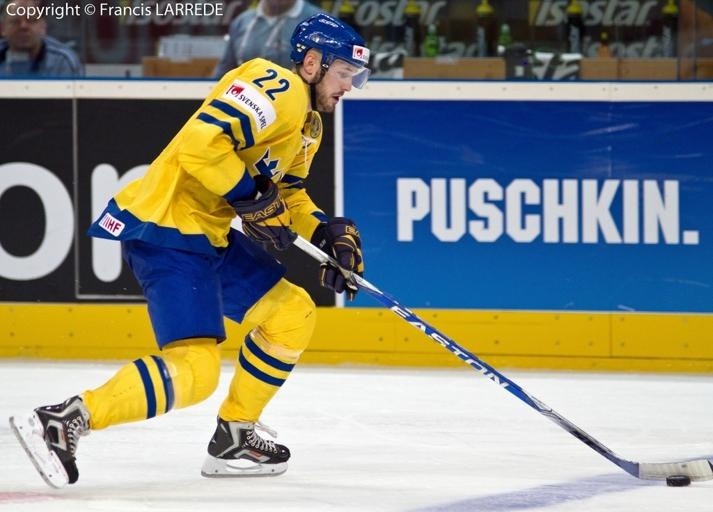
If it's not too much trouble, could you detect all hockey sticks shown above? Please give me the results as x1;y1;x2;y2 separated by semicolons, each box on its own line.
292;233;713;483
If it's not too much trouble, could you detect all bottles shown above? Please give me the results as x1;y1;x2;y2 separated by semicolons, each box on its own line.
496;23;514;57
598;32;611;58
422;23;440;58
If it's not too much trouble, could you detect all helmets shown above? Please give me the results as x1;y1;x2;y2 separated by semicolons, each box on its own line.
289;13;371;75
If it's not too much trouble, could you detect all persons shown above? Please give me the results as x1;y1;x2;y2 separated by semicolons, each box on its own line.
211;0;343;79
8;14;371;491
0;1;84;78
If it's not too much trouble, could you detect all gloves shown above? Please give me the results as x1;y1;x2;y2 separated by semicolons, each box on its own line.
315;212;366;302
228;172;300;253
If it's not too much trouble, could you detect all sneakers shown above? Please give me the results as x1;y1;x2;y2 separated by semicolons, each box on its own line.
32;394;93;485
207;413;291;465
